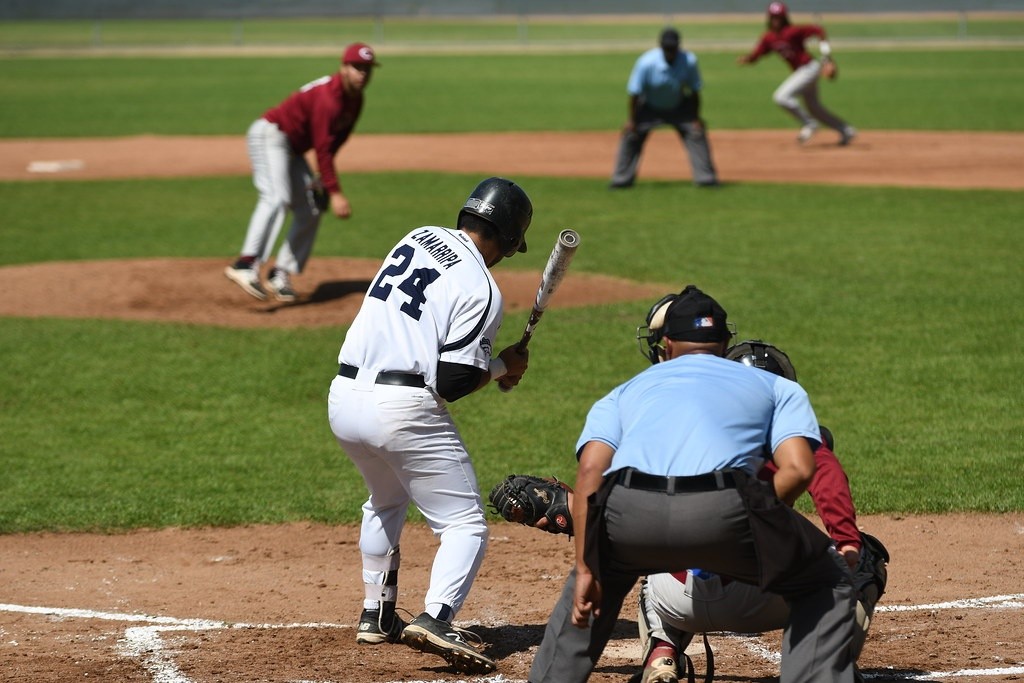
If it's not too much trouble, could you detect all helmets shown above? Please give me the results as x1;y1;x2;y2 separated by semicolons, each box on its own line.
766;2;787;20
456;174;532;254
724;340;798;383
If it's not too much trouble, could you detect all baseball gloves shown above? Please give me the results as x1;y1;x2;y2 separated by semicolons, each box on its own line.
302;173;330;216
820;57;838;79
486;472;572;541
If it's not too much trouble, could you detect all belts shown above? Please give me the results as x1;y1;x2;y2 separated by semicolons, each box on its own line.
339;364;426;388
617;468;737;492
669;570;735;586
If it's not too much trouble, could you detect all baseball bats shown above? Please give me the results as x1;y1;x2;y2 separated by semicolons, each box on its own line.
497;229;580;393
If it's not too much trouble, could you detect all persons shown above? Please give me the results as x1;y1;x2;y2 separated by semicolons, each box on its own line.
224;41;382;304
607;25;727;187
635;335;891;683
524;285;860;683
326;176;533;672
737;0;857;147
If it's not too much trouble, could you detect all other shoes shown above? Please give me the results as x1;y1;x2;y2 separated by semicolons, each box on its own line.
838;128;855;147
796;120;819;141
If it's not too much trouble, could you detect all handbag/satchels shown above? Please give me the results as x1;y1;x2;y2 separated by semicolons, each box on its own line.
740;484;834;594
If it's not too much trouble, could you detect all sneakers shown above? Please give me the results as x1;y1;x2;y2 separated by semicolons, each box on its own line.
641;645;681;683
401;609;498;673
224;257;268;301
262;266;296;302
356;608;413;644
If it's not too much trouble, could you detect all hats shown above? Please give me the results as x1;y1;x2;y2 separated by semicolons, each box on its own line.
648;300;732;342
343;43;381;64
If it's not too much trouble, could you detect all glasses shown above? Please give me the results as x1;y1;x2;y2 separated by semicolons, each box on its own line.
351;63;372;72
